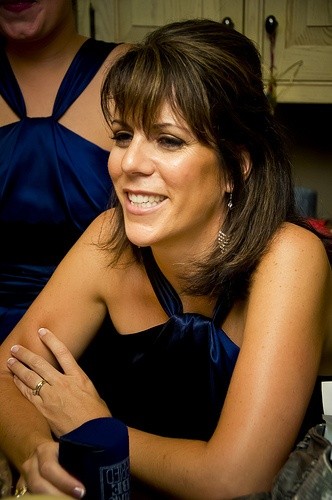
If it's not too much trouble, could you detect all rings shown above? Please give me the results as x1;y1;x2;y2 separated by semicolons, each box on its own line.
33;380;47;394
16;487;28;498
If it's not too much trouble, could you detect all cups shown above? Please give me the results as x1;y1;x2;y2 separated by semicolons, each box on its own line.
293;445;332;500
59;417;129;500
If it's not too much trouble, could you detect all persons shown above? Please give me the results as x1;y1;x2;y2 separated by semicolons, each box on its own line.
0;19;332;500
0;0;140;343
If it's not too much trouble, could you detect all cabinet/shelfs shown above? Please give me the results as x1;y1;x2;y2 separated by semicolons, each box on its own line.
77;0;332;104
